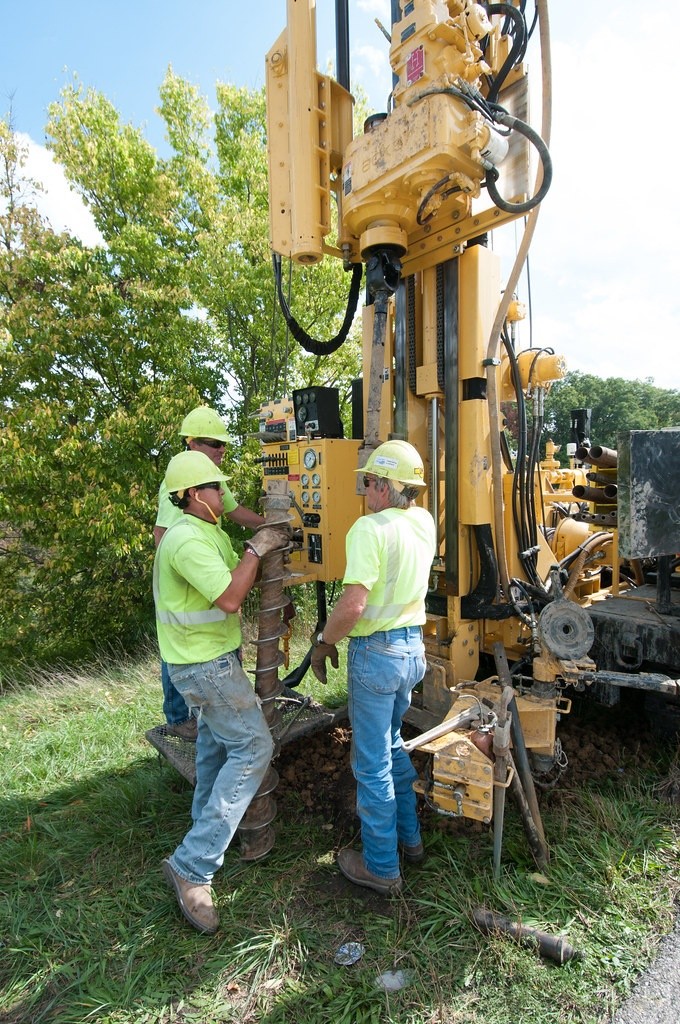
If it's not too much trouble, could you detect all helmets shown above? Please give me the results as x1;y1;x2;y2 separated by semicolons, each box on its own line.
177;407;236;442
165;450;233;493
352;439;426;487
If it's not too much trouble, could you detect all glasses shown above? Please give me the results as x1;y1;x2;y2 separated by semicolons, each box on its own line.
201;440;226;449
362;476;376;488
196;481;221;491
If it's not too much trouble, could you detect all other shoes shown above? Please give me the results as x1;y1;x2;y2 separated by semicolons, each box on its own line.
338;848;404;896
398;842;424;863
160;862;221;935
166;719;198;742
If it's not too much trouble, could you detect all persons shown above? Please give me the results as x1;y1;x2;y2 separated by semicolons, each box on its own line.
309;441;438;894
153;406;293;934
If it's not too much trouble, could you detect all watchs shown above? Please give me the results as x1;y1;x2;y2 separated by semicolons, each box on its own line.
317;633;335;646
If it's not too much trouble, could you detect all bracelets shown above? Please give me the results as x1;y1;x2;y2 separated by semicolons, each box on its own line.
245;549;259;558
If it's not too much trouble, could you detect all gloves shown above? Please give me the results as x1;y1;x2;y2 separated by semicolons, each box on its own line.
243;522;294;560
310;632;339;685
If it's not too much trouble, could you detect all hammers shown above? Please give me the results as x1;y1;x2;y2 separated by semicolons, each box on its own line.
275;697;303;710
